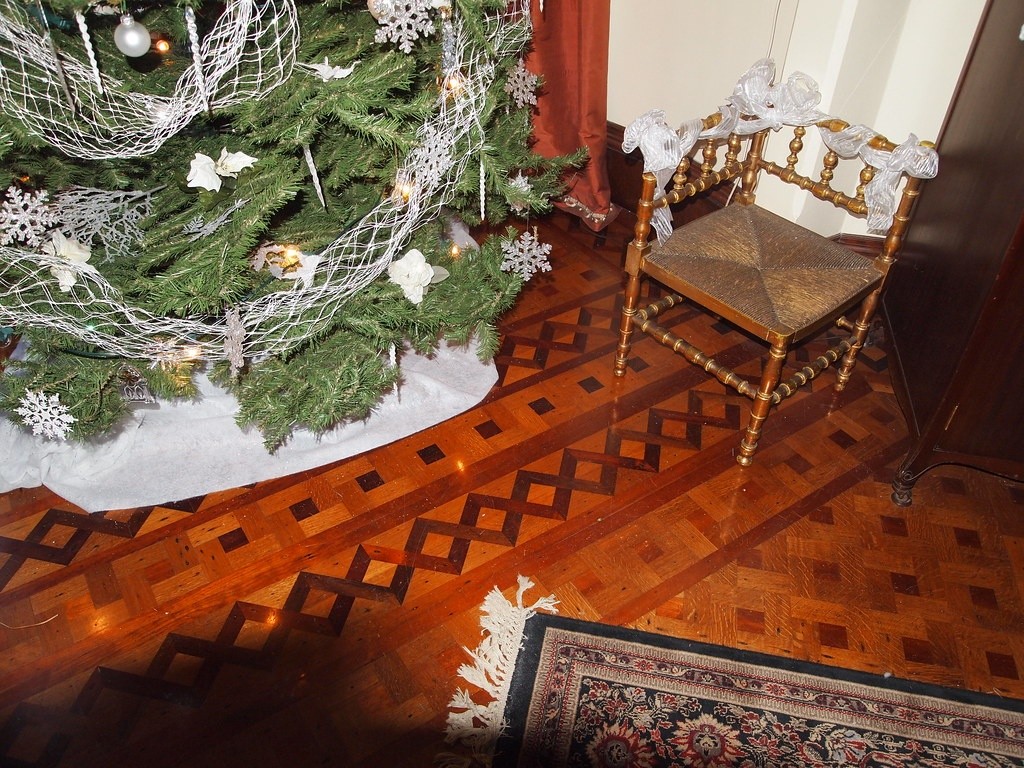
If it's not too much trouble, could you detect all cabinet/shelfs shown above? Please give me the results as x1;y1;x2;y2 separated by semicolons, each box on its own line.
837;0;1024;508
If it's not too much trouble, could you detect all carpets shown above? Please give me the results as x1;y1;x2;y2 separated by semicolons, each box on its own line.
434;574;1024;768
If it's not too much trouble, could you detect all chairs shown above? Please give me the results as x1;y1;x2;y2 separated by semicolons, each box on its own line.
611;58;935;462
